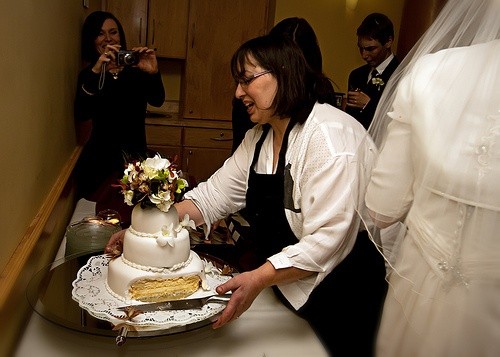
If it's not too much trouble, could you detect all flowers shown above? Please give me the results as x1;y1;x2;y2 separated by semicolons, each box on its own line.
178;215;197;233
110;155;189;214
203;258;222;277
158;223;176;248
372;77;384;91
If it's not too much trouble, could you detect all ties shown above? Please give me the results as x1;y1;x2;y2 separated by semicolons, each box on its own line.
368;69;379;90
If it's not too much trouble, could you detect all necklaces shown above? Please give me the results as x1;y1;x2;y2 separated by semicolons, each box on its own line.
111;68;118;79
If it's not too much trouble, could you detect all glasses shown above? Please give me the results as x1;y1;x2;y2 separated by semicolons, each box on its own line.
240;68;273;86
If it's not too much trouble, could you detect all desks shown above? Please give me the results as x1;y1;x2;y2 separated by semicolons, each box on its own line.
15;198;328;356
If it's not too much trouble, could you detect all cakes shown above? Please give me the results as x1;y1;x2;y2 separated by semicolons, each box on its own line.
108;155;210;305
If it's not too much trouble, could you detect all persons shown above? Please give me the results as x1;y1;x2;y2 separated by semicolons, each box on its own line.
346;0;500;357
347;12;403;132
106;35;388;357
73;10;165;203
230;17;344;156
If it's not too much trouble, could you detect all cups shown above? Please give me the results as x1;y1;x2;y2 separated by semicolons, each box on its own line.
97;209;121;228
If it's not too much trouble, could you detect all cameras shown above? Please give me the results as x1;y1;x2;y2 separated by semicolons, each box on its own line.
116;49;141;66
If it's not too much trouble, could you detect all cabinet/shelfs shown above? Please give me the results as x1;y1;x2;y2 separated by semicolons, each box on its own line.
104;0;277;192
